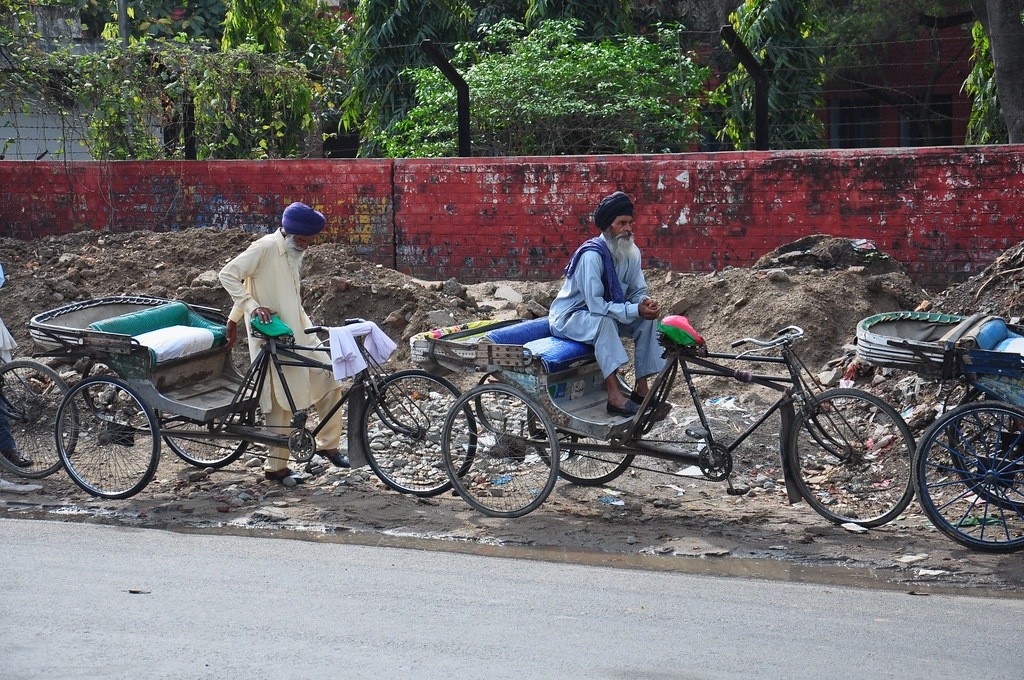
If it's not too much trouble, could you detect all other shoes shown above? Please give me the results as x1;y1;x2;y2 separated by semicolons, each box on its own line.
630;391;658;407
4;449;34;467
315;448;350;468
265;468;303;485
607;398;641;418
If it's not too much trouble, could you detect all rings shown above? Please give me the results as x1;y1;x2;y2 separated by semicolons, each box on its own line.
262;312;264;314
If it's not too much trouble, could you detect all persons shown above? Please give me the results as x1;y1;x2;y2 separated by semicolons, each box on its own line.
219;201;351;484
548;192;672;418
0;262;34;469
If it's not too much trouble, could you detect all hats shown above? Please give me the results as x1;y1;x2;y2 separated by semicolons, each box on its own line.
594;191;635;232
282;201;326;236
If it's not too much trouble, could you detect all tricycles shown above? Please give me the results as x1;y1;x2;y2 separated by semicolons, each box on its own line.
26;295;480;500
0;284;81;479
410;315;917;531
812;309;1024;554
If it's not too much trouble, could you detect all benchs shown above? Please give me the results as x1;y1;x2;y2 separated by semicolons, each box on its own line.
88;300;227;371
961;316;1024;364
482;315;600;385
